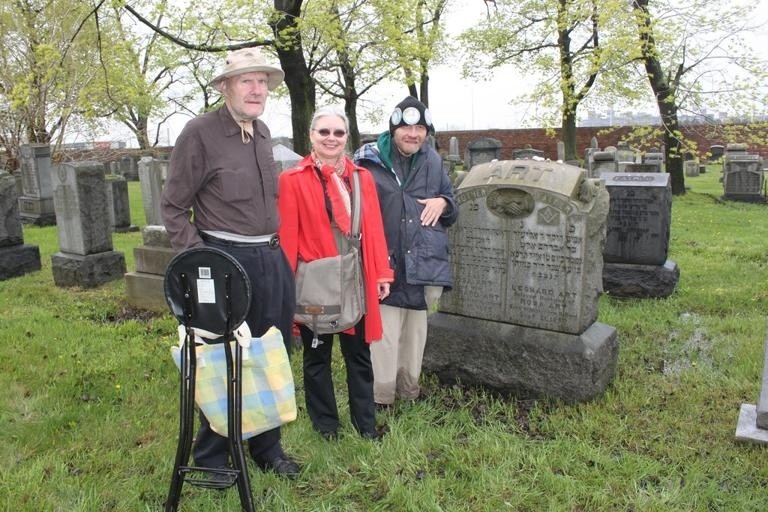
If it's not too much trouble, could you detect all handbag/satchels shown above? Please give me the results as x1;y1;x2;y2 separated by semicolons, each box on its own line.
170;321;297;440
291;247;366;349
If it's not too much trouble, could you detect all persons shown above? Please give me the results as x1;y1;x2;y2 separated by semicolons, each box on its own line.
353;97;459;410
161;46;300;487
279;105;395;442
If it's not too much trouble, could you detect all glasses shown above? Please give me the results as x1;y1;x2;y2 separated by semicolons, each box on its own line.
313;128;346;138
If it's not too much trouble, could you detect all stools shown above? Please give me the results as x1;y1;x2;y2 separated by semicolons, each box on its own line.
164;247;256;512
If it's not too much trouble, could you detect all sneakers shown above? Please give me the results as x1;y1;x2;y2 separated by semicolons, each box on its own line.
361;430;380;442
204;463;237;487
261;451;300;481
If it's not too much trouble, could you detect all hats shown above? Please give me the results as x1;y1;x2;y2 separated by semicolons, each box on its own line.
390;96;431;136
207;48;286;91
